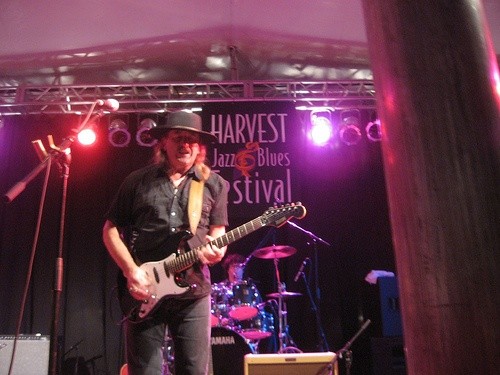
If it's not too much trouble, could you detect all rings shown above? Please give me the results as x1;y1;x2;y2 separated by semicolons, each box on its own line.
130;286;134;293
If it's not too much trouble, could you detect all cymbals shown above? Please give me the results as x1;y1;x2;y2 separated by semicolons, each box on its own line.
265;291;305;296
252;245;298;259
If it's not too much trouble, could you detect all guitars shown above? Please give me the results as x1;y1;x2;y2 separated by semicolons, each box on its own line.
138;202;306;321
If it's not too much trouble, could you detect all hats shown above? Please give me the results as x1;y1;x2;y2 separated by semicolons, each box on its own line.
149;111;215;144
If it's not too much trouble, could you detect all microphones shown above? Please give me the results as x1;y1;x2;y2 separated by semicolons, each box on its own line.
92;98;120;121
293;258;307;282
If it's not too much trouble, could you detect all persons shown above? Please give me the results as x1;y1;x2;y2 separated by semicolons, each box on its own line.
103;112;228;375
209;254;274;354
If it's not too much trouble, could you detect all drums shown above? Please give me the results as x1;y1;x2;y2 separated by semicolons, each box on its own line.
234;311;274;338
229;280;260;321
211;283;231;317
211;325;253;375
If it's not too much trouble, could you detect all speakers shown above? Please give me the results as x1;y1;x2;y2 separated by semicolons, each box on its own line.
352;337;407;375
244;352;338;375
0;335;51;375
370;276;402;336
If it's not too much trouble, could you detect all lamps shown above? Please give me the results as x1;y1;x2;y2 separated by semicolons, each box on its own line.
135;118;158;147
302;116;333;145
107;118;130;147
338;116;362;145
78;122;97;145
366;118;383;143
